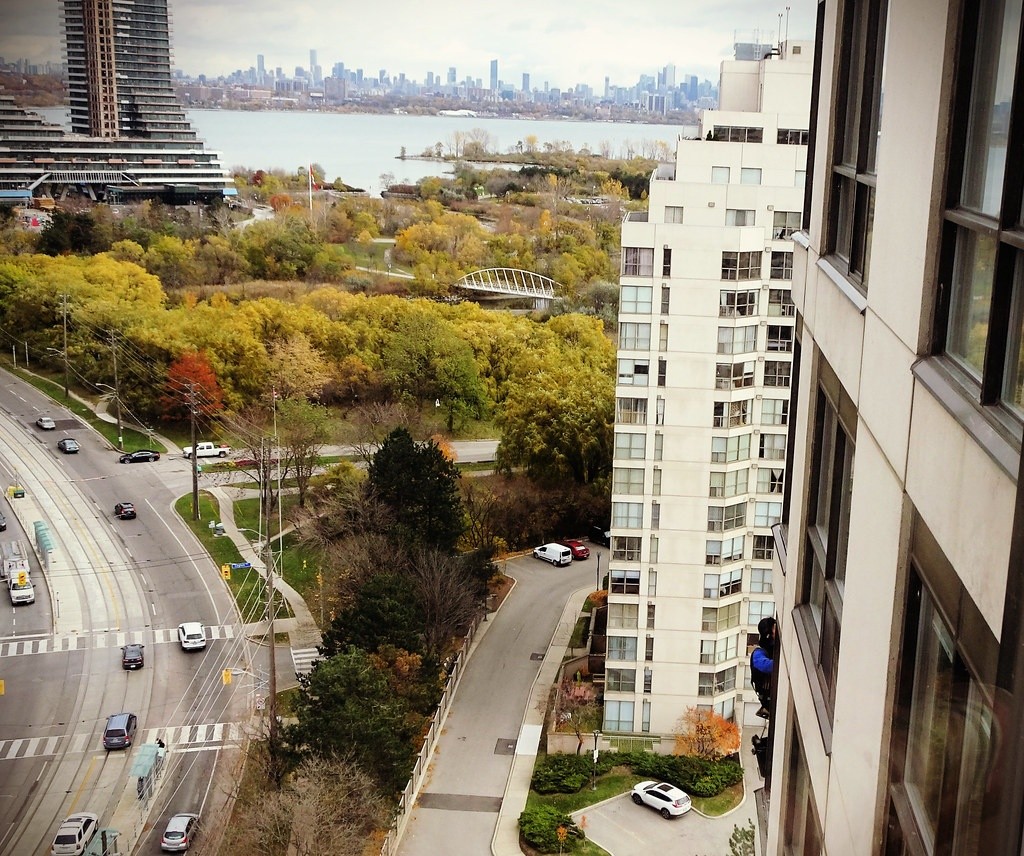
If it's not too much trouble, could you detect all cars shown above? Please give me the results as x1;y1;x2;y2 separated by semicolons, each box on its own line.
114;502;137;520
51;811;99;855
160;812;199;851
36;416;55;429
562;541;590;559
0;512;7;531
58;438;80;454
120;643;145;670
103;712;137;749
118;450;160;464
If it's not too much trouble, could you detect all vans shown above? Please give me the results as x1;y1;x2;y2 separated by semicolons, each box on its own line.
533;543;573;567
7;569;36;605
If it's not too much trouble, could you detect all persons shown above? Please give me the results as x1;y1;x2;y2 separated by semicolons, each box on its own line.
749;616;776;715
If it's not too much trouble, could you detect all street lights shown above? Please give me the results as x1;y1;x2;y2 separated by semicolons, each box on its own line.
95;383;124;449
231;527;289;780
591;729;600;790
46;347;70;397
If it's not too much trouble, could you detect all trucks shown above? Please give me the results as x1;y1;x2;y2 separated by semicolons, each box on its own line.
1;540;31;579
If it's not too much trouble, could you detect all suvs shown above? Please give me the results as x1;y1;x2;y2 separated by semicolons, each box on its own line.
178;621;207;651
631;780;693;820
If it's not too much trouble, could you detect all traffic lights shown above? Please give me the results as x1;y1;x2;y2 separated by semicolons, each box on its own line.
222;567;230;581
18;572;27;586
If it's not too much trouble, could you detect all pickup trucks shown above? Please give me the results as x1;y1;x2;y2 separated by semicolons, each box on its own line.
183;442;232;460
